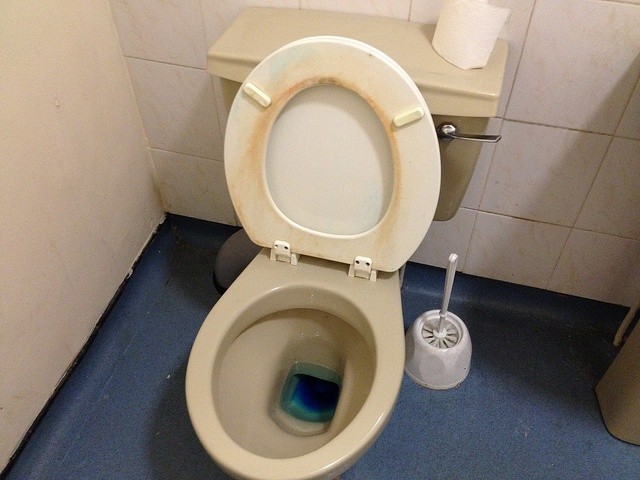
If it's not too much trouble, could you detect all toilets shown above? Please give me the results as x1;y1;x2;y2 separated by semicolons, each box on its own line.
184;7;510;480
